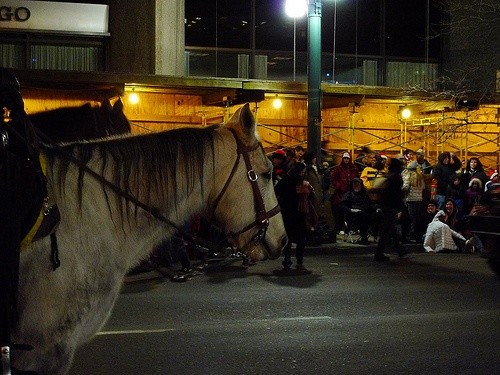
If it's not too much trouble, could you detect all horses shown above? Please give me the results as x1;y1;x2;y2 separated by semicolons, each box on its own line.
0;101;292;375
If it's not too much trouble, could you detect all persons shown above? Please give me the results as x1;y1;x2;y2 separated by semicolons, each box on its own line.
298;139;385;245
141;219;229;282
0;66;47;349
374;157;412;262
267;146;305;187
400;149;500;254
274;162;315;274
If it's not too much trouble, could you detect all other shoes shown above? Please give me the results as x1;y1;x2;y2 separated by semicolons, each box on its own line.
297;268;312;274
479;248;488;258
282;264;290;272
181;266;204;277
361;237;370;244
168;272;182;281
466;237;475;253
400;238;411;244
375;252;390;262
398;248;413;257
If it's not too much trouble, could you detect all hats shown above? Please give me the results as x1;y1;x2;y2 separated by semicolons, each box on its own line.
303;152;315;161
342;153;350;158
468;177;483;190
